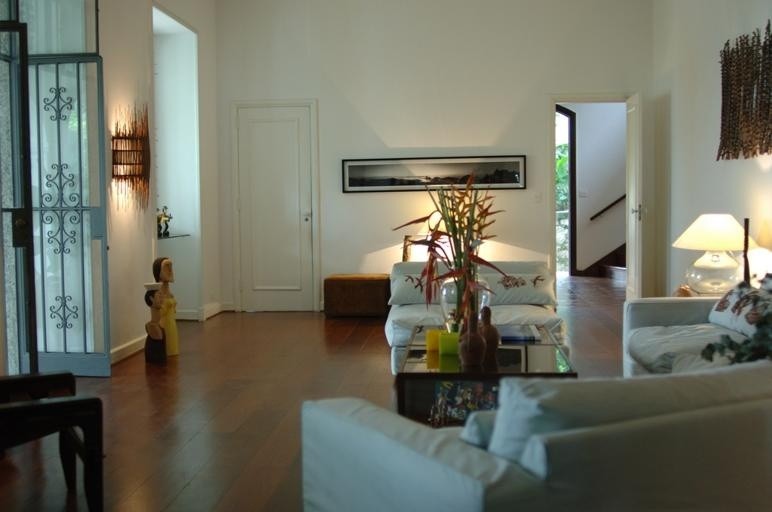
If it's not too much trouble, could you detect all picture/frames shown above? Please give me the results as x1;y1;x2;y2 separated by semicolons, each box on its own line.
340;153;529;195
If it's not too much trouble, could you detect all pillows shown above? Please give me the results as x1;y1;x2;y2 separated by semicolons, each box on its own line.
387;273;558;308
708;282;771;336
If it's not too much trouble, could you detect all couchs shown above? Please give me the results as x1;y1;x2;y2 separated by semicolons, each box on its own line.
385;255;568;356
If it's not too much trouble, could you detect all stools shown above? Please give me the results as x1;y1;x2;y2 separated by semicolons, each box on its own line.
321;270;390;319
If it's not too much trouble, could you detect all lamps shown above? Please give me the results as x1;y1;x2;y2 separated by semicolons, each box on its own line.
672;212;757;296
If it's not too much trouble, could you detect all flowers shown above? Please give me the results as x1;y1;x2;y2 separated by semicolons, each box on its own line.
394;174;509;326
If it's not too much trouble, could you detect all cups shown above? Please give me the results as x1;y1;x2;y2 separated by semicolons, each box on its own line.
438;334;461;355
425;330;447;352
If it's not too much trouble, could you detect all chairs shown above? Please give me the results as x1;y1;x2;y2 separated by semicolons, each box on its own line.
619;274;772;375
301;346;772;511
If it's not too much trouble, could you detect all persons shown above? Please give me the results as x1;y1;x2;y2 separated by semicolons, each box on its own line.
151;256;180;359
157;207;163;237
162;205;173;237
143;288;170;366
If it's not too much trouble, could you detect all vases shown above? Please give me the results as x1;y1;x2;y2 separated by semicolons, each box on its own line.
438;272;493;324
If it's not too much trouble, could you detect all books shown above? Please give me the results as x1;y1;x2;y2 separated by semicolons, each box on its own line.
410;323;455;351
492;321;542;342
